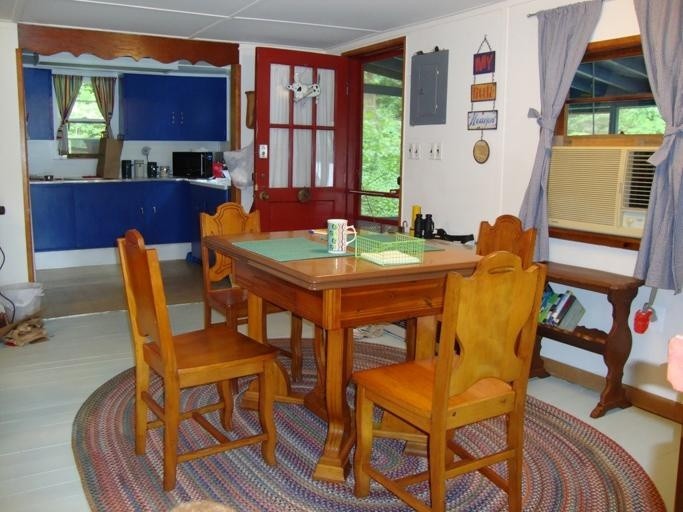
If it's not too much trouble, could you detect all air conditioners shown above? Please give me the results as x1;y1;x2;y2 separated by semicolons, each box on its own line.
545;145;663;240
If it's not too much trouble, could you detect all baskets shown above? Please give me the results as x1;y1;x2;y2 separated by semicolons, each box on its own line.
355;231;425;267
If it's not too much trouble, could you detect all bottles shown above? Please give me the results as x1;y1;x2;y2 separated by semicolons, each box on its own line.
122;159;168;179
414;213;434;238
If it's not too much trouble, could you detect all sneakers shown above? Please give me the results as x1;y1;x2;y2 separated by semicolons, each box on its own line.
352;324;384;339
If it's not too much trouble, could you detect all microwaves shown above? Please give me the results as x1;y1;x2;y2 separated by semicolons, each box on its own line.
173;151;223;179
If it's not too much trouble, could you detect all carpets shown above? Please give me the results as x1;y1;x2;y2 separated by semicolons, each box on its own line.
72;337;668;511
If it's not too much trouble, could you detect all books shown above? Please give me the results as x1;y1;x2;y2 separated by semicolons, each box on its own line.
537;284;586;333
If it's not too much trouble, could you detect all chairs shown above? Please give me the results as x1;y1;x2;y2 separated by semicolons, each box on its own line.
350;247;548;510
115;227;281;496
199;200;308;385
468;210;539;268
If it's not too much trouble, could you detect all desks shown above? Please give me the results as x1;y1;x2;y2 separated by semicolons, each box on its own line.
201;223;485;486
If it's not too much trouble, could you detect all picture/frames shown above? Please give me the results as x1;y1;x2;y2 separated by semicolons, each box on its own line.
467;50;497;130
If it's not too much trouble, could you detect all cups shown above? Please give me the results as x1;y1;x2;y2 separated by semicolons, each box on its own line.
327;218;357;255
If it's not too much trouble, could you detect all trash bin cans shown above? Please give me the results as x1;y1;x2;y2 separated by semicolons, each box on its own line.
0;282;43;324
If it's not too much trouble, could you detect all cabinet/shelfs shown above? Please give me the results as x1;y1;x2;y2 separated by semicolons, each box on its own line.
22;68;55;140
119;74;226;142
28;182;111;252
111;182;230;247
530;258;647;419
